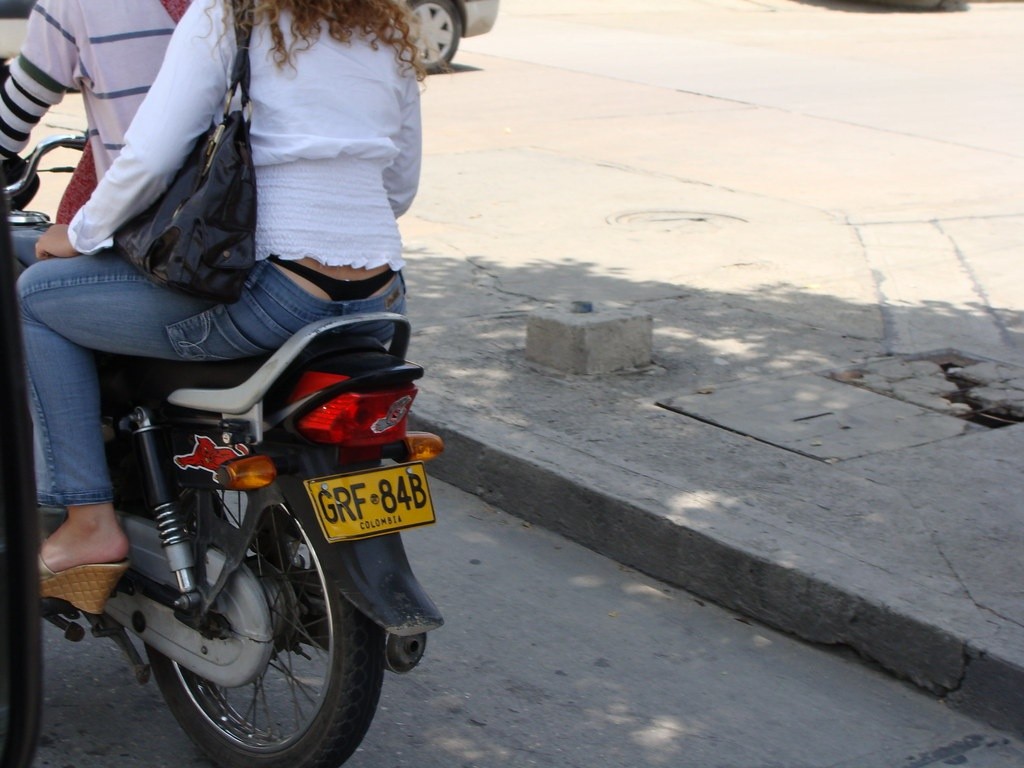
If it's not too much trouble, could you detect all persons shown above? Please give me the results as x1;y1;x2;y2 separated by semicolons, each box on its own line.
17;1;423;575
0;1;245;199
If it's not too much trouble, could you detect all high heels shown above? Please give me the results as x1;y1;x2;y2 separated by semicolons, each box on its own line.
34;543;132;615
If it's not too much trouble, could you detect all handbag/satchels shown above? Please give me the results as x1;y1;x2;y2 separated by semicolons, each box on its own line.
111;0;261;302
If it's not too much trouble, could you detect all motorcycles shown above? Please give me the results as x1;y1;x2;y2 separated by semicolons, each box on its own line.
4;128;448;768
